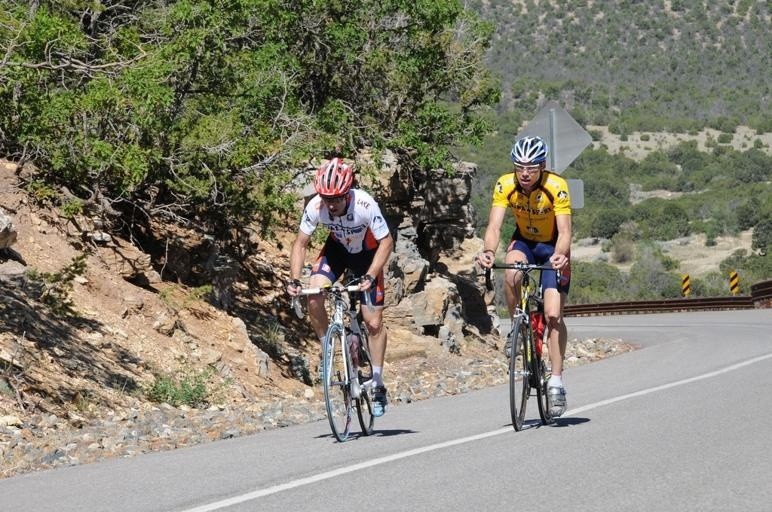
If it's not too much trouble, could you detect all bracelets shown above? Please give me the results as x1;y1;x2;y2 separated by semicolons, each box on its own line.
483;249;497;257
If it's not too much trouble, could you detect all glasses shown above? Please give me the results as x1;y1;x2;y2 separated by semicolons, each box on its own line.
327;197;343;203
514;166;539;172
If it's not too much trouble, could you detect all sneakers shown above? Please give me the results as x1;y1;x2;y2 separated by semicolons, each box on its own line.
546;385;567;416
368;385;386;417
503;333;523;357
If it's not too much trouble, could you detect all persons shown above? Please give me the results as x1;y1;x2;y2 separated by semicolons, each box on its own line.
285;157;394;417
477;135;573;418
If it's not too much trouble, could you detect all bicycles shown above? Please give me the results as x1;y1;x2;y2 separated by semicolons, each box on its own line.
480;257;573;431
289;280;396;440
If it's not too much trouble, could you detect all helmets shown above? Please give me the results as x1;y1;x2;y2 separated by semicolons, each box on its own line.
315;157;353;198
510;136;548;167
